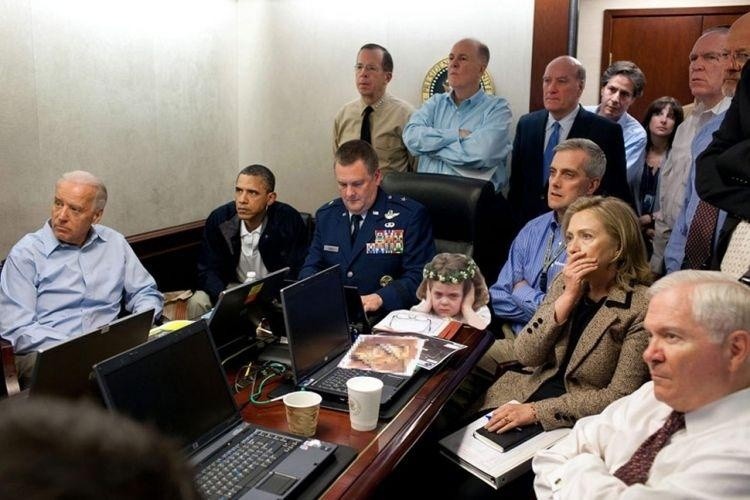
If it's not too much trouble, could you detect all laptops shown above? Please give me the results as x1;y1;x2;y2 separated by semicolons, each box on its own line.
280;265;422;407
25;309;156;396
282;280;378;330
93;320;338;499
207;266;292;329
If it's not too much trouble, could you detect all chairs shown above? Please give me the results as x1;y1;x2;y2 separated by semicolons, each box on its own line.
299;211;312;235
379;174;501;286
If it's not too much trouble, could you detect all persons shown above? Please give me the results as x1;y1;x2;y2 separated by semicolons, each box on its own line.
649;30;732;277
401;38;514;195
510;56;628;195
582;60;647;196
0;169;165;392
409;252;493;331
294;139;437;318
529;268;750;500
638;96;683;245
185;163;307;323
425;195;655;435
663;13;750;282
478;137;606;382
329;42;421;174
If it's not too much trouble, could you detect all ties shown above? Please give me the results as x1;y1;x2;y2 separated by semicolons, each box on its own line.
542;122;561;187
720;221;750;280
612;407;688;488
360;106;375;150
681;198;720;269
349;214;365;243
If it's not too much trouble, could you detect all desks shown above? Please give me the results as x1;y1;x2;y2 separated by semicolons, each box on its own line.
100;290;496;500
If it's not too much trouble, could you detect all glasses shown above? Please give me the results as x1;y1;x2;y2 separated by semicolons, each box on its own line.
351;62;389;74
388;310;432;332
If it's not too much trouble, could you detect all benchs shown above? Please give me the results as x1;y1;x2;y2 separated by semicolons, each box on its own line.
0;218;209;395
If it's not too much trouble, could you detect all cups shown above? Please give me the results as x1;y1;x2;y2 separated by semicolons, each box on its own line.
345;374;385;432
281;388;326;439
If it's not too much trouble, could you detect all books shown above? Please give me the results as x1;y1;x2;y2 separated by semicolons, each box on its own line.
444;398;573;491
473;419;543;452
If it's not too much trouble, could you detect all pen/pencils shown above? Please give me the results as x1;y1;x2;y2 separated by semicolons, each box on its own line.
555;262;565;266
486;416;522;431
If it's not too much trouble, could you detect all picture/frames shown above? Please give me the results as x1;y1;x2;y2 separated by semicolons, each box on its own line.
421;57;495;104
